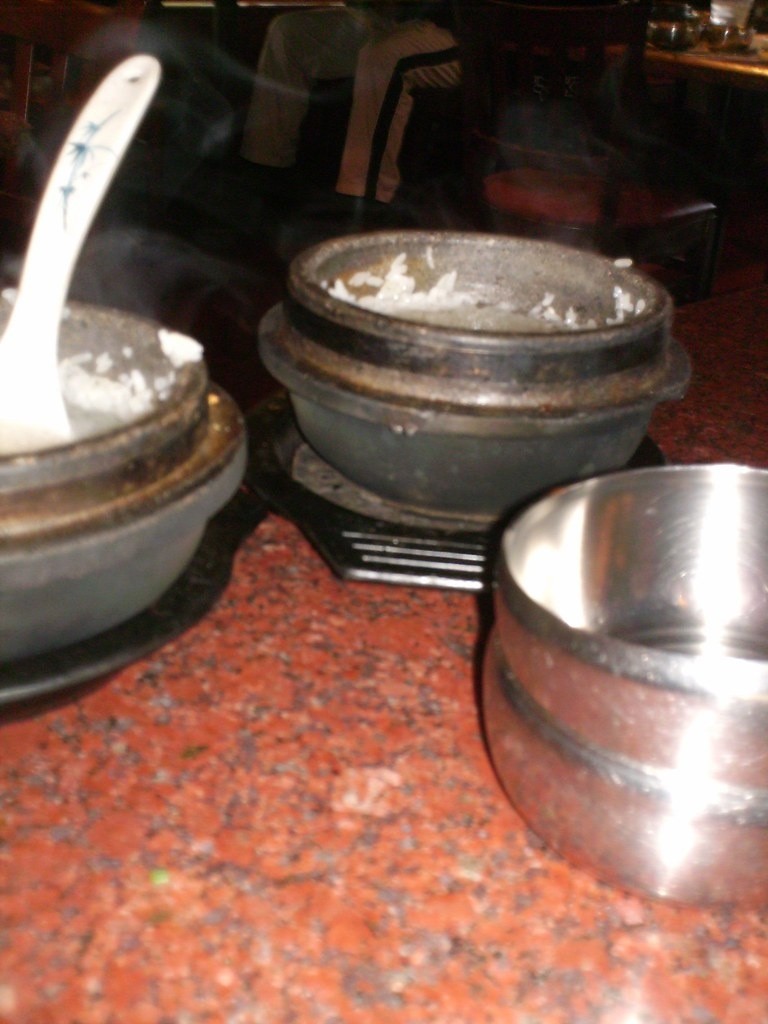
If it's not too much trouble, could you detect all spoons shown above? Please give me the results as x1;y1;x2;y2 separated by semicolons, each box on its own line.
0;53;160;456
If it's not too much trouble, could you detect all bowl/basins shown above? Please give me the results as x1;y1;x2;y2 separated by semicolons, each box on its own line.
0;287;248;674
706;25;753;52
257;228;691;510
480;462;768;901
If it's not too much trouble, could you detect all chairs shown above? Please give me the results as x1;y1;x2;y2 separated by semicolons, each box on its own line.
480;0;744;302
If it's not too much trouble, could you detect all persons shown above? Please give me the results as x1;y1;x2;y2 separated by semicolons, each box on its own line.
237;0;465;203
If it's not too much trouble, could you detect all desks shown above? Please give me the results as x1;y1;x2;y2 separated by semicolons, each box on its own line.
0;236;767;1024
641;31;767;93
